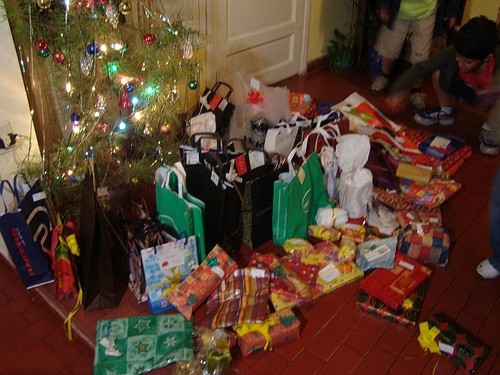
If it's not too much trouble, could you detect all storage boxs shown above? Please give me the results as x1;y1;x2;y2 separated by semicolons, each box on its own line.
236;177;488;375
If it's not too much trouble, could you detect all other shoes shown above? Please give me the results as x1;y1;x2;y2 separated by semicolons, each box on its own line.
370;75;389;96
409;92;426;110
476;258;500;279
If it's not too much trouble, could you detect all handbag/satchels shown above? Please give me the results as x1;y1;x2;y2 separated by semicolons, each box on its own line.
298;107;349;166
264;122;299;158
128;132;373;314
0;173;54;289
77;157;132;311
192;81;235;137
52;222;79;312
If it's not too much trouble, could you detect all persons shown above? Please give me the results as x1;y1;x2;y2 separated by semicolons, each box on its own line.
414;15;500;156
369;0;467;110
476;168;500;279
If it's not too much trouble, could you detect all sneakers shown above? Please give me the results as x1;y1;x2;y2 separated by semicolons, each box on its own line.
414;107;456;125
479;130;499;155
